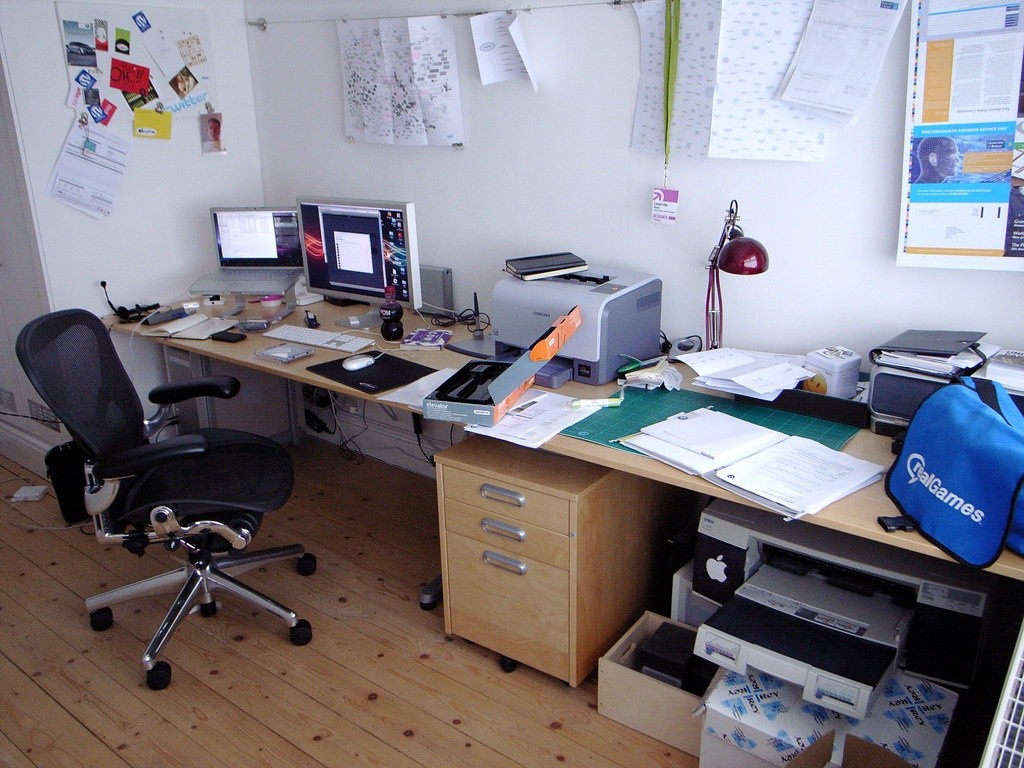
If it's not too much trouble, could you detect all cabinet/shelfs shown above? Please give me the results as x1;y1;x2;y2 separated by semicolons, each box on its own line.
434;434;660;689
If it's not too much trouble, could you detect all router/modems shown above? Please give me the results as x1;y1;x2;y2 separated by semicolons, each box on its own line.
13;485;47;502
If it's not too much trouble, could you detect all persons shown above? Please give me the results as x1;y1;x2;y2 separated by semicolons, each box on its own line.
201;118;227;153
176;73;196;95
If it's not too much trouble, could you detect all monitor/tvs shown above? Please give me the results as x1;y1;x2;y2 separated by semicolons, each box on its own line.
296;195;422;330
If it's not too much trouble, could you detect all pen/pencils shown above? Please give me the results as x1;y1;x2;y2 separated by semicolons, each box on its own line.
473;292;480;330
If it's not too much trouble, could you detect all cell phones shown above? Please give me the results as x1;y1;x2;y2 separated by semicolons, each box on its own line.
210;331;247;343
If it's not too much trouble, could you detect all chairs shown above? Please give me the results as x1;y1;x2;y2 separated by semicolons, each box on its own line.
15;308;317;689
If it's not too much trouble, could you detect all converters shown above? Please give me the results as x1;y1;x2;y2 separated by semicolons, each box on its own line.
305;409;327;433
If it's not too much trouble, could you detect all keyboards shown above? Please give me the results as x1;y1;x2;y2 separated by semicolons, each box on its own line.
262;325;375;354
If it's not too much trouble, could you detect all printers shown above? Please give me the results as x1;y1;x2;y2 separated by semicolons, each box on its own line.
485;263;663;388
669;499;994;721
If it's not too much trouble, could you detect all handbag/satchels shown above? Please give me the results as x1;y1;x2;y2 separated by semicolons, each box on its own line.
878;367;1024;571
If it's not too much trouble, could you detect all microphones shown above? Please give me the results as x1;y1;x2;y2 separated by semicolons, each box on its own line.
100;281;118;313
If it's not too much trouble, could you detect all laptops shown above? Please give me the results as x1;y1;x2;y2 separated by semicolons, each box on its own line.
189;207;305;296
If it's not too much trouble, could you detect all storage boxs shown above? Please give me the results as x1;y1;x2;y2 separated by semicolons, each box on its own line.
598;610;959;768
423;305;583;427
802;345;861;400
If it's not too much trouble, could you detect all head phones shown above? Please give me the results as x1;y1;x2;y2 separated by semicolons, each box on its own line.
116;302;160;320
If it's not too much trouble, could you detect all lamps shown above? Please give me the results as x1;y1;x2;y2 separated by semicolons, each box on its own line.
705;200;769;350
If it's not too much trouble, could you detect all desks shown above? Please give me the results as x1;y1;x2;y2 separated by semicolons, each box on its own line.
99;288;1024;582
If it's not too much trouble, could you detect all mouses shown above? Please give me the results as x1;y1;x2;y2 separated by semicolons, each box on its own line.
342;355;375;371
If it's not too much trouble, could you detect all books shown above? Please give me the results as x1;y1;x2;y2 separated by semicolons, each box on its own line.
505;252;590;281
